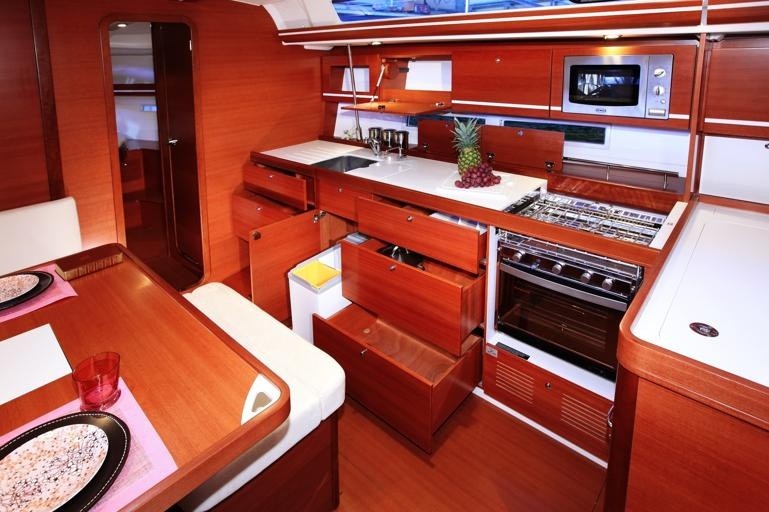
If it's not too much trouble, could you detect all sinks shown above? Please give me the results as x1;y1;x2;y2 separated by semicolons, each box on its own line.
310;154;382;173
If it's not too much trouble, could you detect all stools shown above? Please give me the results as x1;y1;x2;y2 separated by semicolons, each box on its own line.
176;282;346;512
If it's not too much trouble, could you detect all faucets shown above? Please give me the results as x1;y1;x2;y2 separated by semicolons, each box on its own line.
364;138;381;156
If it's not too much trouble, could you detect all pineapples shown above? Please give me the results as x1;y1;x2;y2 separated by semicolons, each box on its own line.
449;115;483;176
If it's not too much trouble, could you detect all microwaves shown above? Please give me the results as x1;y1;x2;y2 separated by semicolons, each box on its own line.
563;54;676;122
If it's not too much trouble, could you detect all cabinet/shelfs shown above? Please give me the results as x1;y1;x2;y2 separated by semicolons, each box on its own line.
698;38;769;140
452;42;553;118
313;194;488;455
341;45;451;114
318;176;386;224
231;163;321;323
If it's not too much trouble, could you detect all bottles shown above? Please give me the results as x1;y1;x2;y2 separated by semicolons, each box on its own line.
368;127;409;150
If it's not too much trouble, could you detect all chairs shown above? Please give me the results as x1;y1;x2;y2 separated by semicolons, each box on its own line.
0;197;83;277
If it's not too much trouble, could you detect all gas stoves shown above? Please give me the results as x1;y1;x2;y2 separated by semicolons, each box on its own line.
519;192;666;252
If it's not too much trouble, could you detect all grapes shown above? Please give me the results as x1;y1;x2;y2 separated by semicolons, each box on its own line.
455;163;501;189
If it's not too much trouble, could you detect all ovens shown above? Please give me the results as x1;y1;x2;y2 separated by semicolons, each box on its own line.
496;266;627;384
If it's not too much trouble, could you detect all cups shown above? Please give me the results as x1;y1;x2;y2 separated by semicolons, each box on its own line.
70;352;120;416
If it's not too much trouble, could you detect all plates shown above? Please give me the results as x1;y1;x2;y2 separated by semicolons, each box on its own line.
1;269;54;313
0;411;130;510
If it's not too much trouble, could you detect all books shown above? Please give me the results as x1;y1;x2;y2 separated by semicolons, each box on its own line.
289;259;343;295
